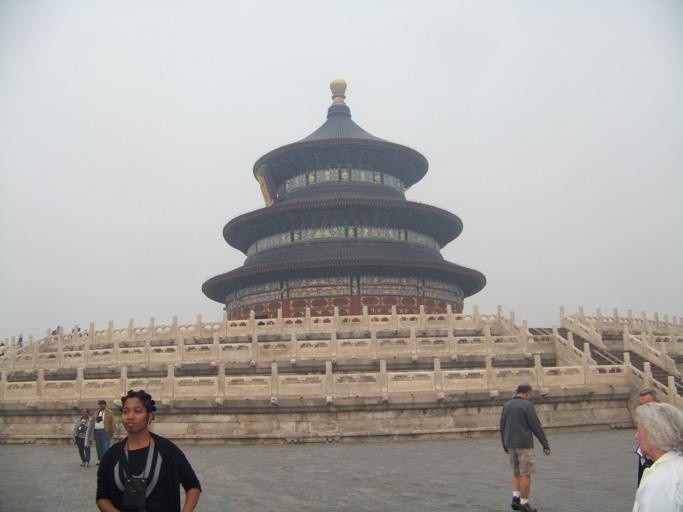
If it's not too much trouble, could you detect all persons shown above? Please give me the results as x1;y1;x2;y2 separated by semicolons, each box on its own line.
92;400;116;465
51;326;88;339
97;389;202;512
18;337;23;353
629;401;683;512
500;382;550;511
72;408;94;467
635;389;661;487
0;342;5;356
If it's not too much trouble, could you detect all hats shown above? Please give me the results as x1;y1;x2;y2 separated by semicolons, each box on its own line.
98;400;107;404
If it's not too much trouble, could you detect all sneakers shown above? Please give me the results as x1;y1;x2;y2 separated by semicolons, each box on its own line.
511;497;537;512
95;459;101;466
80;461;89;467
